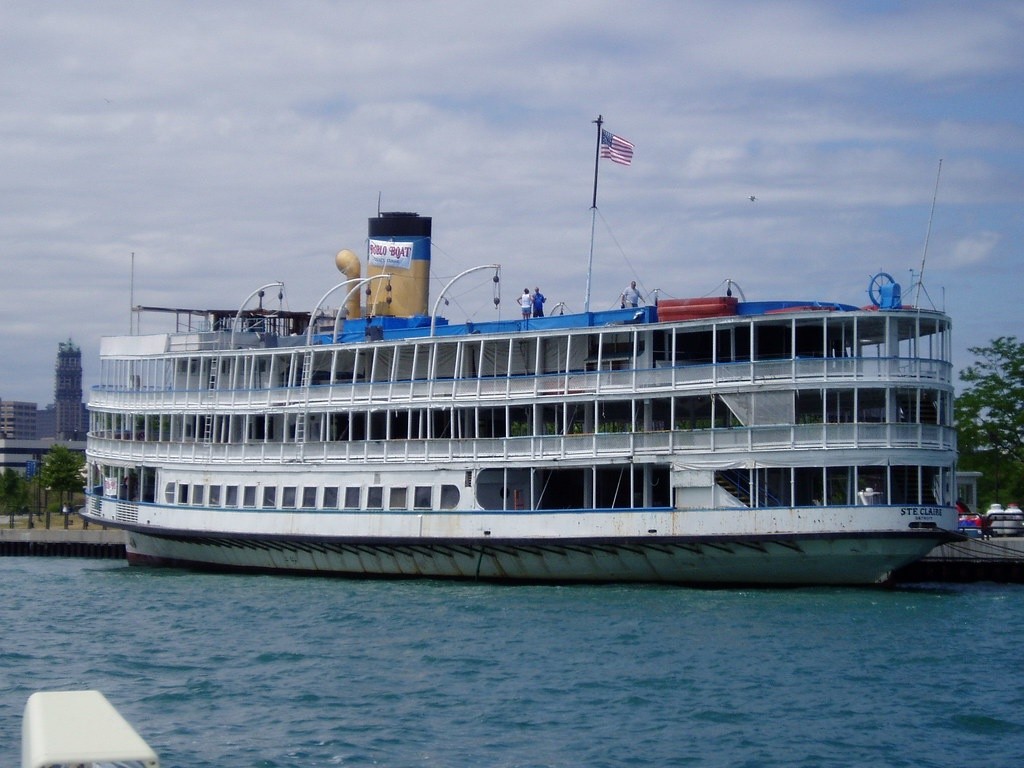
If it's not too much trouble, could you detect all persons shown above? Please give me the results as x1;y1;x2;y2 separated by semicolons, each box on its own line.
517;288;535;319
621;281;645;308
530;288;547;318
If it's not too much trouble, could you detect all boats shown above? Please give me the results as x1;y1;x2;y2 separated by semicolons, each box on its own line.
76;116;970;585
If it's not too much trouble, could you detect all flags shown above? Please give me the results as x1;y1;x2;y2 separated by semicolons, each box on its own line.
600;128;635;166
